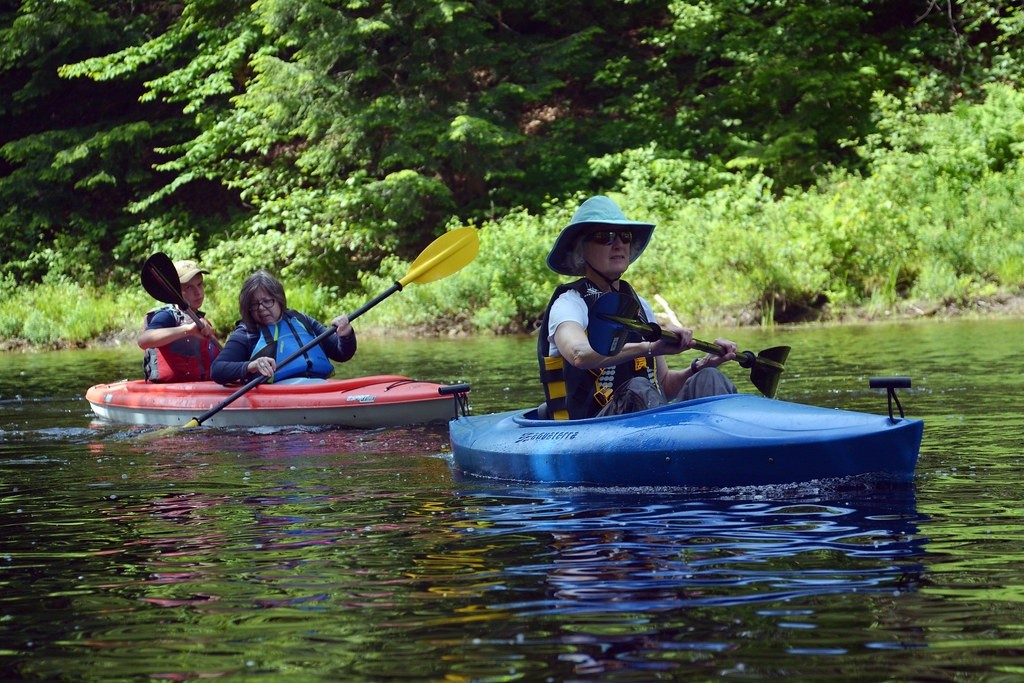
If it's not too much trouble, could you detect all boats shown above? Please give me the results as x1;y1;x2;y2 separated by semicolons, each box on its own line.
85;375;468;429
440;375;925;489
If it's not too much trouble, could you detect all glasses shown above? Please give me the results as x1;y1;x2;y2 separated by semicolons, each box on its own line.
248;299;275;313
584;229;633;246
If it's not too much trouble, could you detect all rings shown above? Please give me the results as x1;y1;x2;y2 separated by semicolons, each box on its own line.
259;360;268;369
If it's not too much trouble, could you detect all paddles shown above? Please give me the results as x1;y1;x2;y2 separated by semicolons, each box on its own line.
176;227;481;430
587;291;791;400
142;252;223;352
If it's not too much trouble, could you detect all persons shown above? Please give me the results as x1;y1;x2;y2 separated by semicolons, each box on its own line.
137;261;224;384
210;271;357;385
537;196;737;420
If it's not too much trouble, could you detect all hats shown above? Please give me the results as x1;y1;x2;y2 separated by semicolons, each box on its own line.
173;260;210;284
547;195;656;276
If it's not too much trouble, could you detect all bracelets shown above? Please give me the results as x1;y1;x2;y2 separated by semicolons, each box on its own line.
647;342;653;358
690;357;701;374
